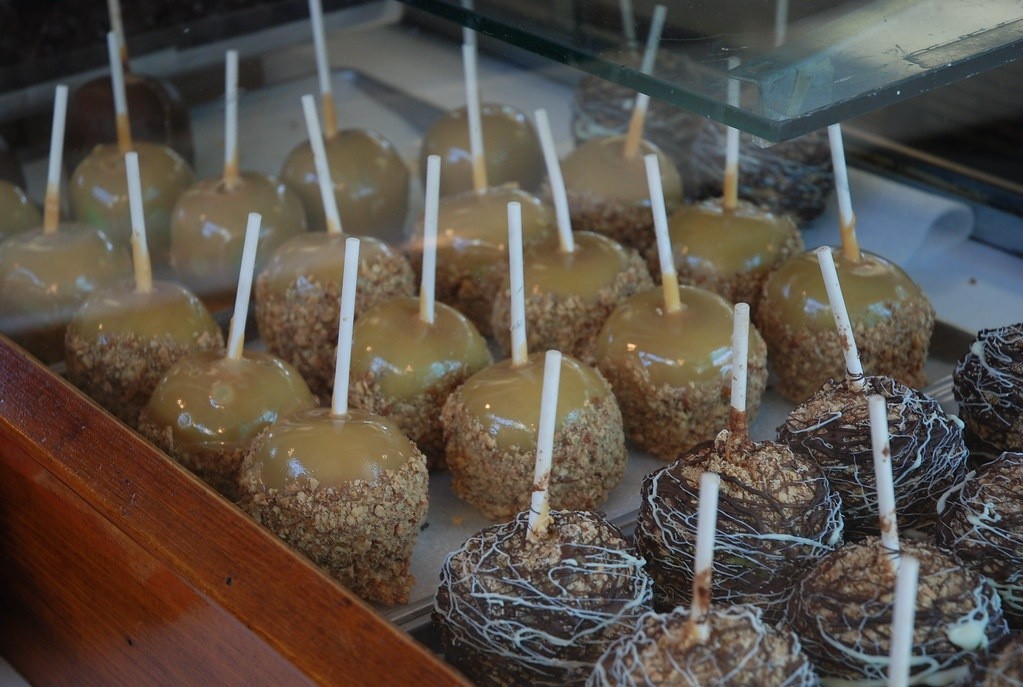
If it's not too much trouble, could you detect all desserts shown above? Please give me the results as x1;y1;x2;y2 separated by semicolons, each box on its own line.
0;69;1023;687
